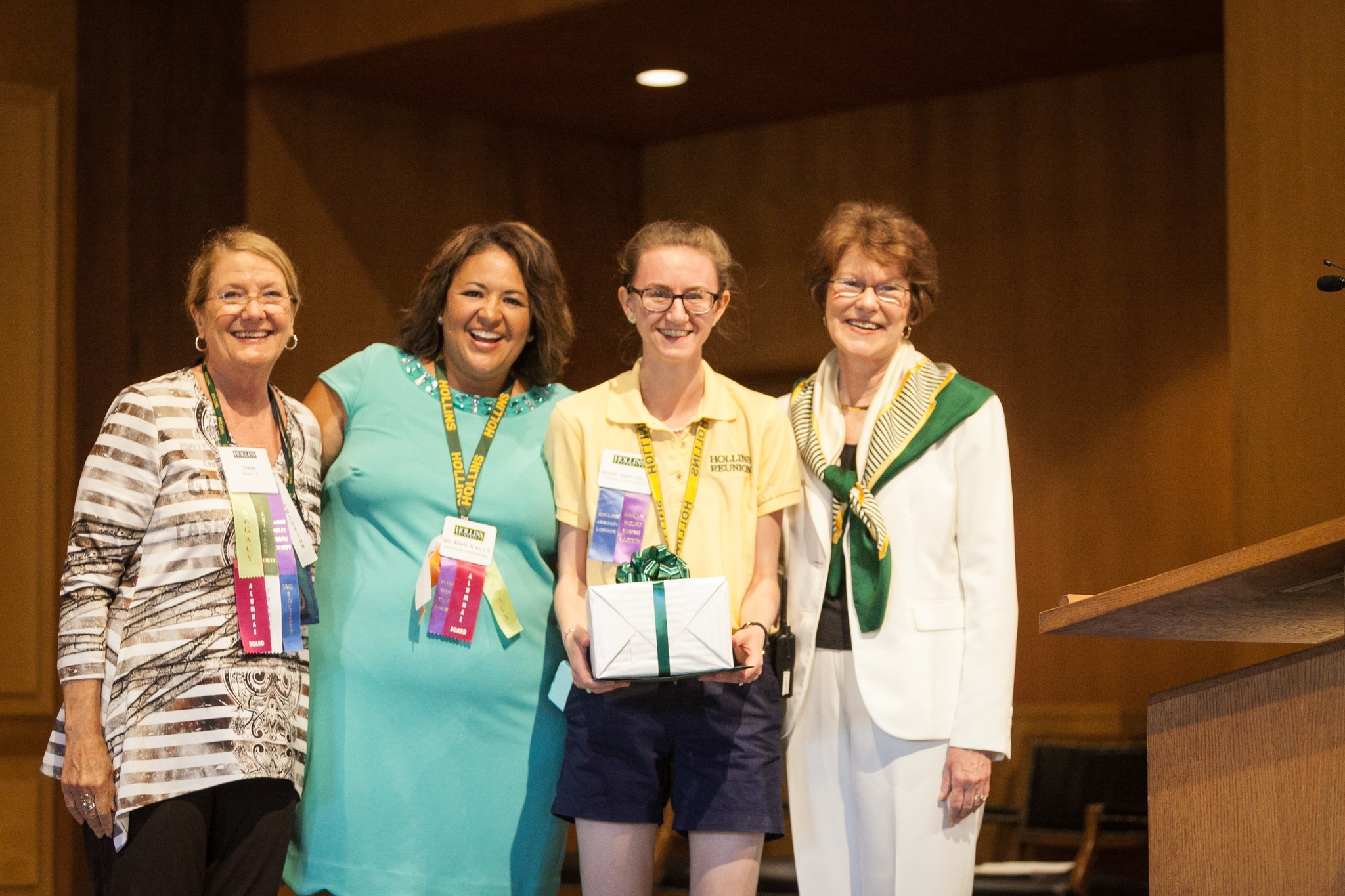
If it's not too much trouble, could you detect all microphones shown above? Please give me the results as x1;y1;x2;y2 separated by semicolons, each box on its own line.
1316;258;1345;295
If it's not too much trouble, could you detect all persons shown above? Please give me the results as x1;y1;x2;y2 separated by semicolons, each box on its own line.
279;216;581;896
775;201;1019;895
33;236;331;894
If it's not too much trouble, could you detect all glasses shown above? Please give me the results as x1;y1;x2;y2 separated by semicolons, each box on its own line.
196;287;294;305
625;284;721;315
826;277;913;303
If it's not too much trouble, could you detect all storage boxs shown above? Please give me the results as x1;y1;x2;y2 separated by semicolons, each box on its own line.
582;576;736;681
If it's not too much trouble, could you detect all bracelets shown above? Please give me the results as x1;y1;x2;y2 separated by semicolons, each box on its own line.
563;627;577;647
739;622;768;654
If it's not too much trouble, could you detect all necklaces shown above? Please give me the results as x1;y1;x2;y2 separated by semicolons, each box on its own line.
635;416;709;559
840;403;868;412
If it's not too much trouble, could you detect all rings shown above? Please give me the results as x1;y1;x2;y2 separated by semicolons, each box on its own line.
976;794;987;802
537;225;804;896
739;680;744;687
586;687;591;694
80;793;95;814
